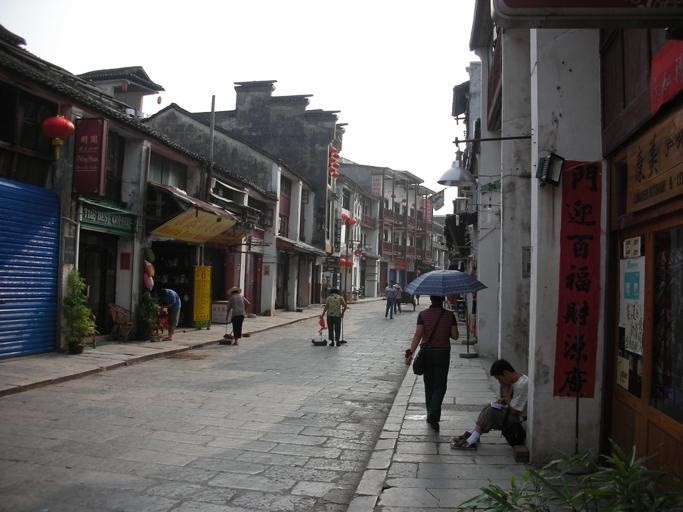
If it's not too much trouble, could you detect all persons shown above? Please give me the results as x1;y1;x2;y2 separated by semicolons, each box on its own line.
449;358;528;450
405;296;458;432
320;286;347;346
156;288;182;341
225;286;250;346
384;280;403;320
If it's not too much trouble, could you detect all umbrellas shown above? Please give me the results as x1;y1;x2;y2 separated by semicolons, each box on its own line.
402;269;489;298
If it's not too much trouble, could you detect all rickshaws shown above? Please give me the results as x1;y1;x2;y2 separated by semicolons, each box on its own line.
380;292;417;313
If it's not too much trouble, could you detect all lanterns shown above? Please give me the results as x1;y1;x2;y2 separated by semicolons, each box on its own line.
337;258;353;275
341;212;356;226
42;113;77;161
328;145;340;181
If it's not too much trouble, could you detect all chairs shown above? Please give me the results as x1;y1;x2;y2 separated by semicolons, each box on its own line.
107;302;139;343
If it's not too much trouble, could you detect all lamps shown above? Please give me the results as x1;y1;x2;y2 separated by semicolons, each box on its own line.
436;130;534;190
536;151;566;189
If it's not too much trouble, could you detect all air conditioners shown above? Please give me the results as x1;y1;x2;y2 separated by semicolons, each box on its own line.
453;196;470;217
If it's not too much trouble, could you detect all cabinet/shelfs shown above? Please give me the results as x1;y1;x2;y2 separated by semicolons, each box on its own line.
209;299;235;326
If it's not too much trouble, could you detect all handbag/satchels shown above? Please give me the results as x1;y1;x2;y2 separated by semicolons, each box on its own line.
412;342;426;376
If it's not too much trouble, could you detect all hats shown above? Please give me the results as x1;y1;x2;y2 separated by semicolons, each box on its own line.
226;286;242;296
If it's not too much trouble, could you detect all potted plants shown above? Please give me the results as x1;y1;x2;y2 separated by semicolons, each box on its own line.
60;266;101;354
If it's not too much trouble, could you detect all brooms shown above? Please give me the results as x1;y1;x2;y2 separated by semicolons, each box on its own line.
223;330;234;341
338;317;347;345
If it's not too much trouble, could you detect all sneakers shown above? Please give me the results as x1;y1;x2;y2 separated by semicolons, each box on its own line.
232;342;239;345
426;418;432;424
452;429;481;443
162;337;172;341
329;342;335;346
336;342;341;346
449;438;479;452
430;421;440;432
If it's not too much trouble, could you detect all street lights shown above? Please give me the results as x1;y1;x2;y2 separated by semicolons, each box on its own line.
342;239;363;305
